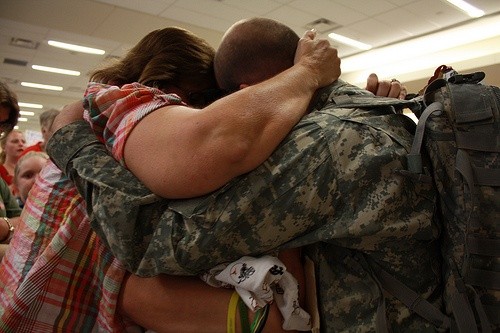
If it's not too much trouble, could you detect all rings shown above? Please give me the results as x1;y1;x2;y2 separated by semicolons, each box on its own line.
391;78;400;83
401;86;407;92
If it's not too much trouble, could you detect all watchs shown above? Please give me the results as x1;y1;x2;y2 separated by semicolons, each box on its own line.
1;216;14;237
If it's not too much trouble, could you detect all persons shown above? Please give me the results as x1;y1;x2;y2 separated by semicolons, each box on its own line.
45;15;500;333
0;26;408;333
0;79;59;333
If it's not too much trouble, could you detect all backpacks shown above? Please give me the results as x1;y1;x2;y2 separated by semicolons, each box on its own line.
303;72;500;333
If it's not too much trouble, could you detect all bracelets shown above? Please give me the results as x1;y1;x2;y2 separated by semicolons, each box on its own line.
226;290;270;333
